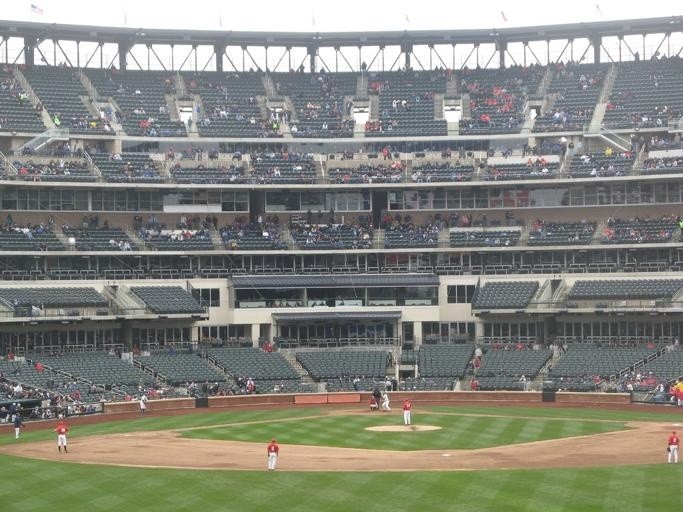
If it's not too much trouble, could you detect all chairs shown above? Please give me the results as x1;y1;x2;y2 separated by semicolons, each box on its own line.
471;280;539;310
296;341;683;393
2;287;109;309
130;285;204;314
566;279;683;300
1;345;301;402
2;60;683;133
232;274;439;286
0;216;683;251
1;151;662;183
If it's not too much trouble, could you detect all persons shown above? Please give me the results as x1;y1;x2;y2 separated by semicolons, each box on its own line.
53;421;70;453
268;439;279;470
267;301;345;307
15;412;25;438
667;430;679;463
